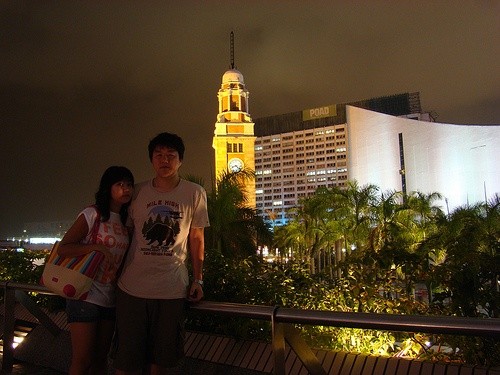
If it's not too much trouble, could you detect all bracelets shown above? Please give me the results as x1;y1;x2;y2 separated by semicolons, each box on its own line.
192;280;204;286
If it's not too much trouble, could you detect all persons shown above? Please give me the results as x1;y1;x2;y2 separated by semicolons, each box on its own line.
57;165;134;375
110;132;210;375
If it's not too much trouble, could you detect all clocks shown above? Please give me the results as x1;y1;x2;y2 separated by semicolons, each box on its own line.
228;158;244;175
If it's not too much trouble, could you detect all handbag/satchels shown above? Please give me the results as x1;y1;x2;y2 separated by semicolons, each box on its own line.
39;204;104;300
